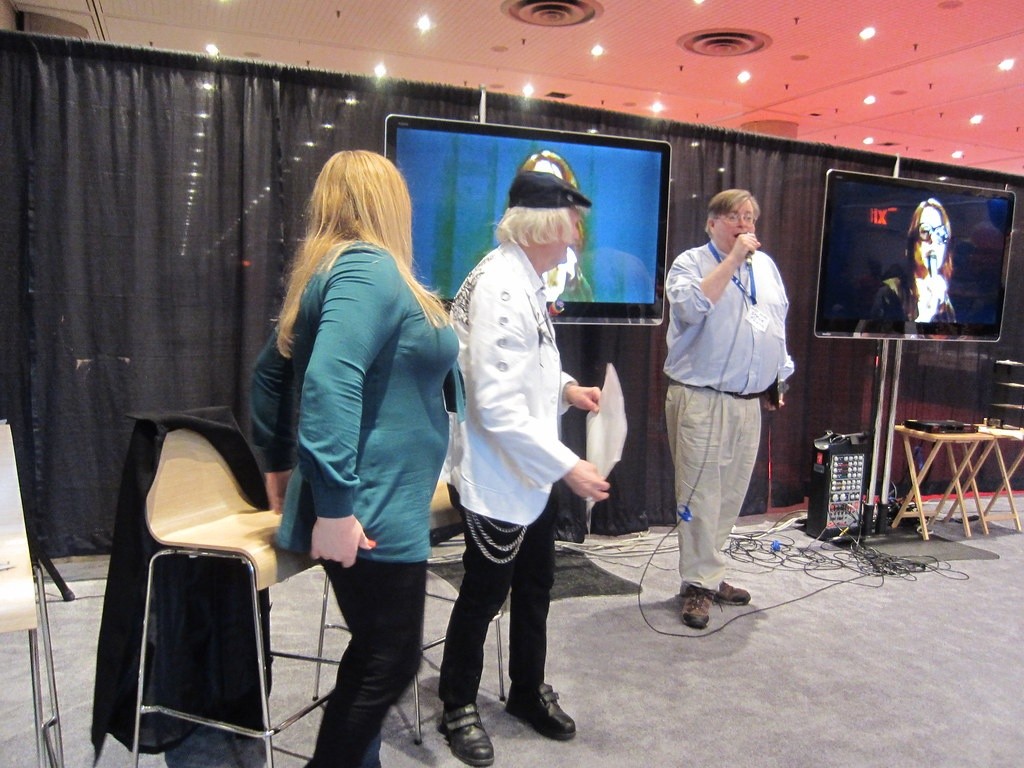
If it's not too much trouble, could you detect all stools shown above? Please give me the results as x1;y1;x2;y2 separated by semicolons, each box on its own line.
943;424;1024;534
891;424;995;541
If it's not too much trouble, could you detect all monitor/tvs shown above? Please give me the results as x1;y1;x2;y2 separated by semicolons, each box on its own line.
384;113;671;324
811;168;1017;343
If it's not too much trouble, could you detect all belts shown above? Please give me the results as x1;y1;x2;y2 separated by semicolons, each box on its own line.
704;386;766;400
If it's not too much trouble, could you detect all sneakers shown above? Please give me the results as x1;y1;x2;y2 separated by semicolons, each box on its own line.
687;580;750;605
679;585;723;629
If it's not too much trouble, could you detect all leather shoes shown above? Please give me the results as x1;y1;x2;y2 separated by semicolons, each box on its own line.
439;701;494;765
504;680;575;741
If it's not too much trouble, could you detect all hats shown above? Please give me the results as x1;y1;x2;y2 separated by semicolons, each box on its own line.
509;169;592;210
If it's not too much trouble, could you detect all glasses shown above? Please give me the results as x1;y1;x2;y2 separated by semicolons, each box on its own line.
916;222;948;244
709;214;758;226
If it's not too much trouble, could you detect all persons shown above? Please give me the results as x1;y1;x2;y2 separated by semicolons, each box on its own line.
865;197;957;323
247;150;460;768
666;188;795;628
437;171;610;767
517;149;596;302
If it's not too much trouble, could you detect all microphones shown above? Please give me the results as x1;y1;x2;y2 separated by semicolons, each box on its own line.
745;251;752;266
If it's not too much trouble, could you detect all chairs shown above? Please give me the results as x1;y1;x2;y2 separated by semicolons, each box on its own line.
91;402;338;768
0;421;64;768
311;480;506;746
